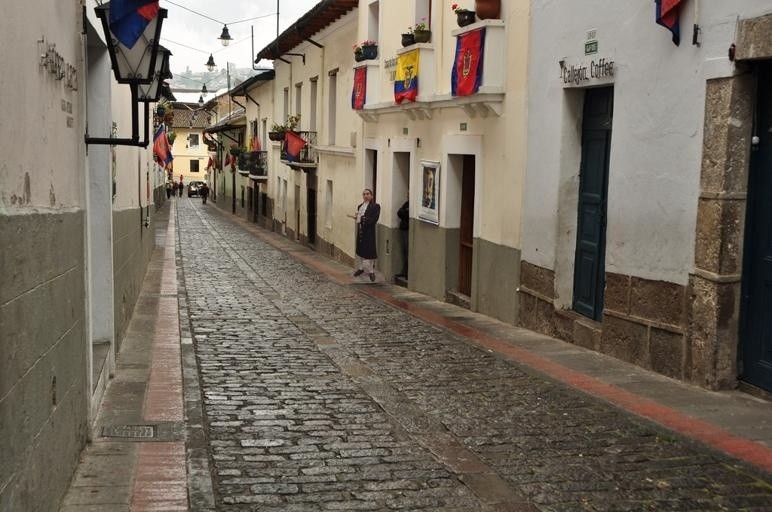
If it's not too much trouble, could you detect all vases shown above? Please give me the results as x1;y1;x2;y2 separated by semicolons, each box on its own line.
414;31;431;44
456;10;477;27
361;46;378;59
401;34;414;47
353;50;368;63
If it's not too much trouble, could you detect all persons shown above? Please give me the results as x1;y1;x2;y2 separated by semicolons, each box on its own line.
395;191;409;280
353;188;381;282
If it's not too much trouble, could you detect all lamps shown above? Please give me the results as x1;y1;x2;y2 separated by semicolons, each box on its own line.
133;34;173;149
85;1;172;147
187;22;233;131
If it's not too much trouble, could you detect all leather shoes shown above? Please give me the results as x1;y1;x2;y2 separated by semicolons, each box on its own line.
354;270;364;276
369;273;375;281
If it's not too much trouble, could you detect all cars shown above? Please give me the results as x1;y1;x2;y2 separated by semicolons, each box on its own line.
186;179;205;199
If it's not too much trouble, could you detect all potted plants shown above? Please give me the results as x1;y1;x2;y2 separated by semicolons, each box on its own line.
267;123;288;140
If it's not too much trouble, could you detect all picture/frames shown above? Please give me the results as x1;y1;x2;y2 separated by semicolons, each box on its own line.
418;159;442;226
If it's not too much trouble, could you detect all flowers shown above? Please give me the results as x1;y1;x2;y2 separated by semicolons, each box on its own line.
450;2;473;10
408;16;432;30
362;38;379;48
351;41;367;49
406;23;418;33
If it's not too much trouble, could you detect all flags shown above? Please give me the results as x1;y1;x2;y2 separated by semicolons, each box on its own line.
394;49;419;106
153;127;174;172
451;27;486;96
351;67;367;110
224;151;236;167
655;1;687;47
208;157;214;169
109;0;158;50
282;130;308;162
